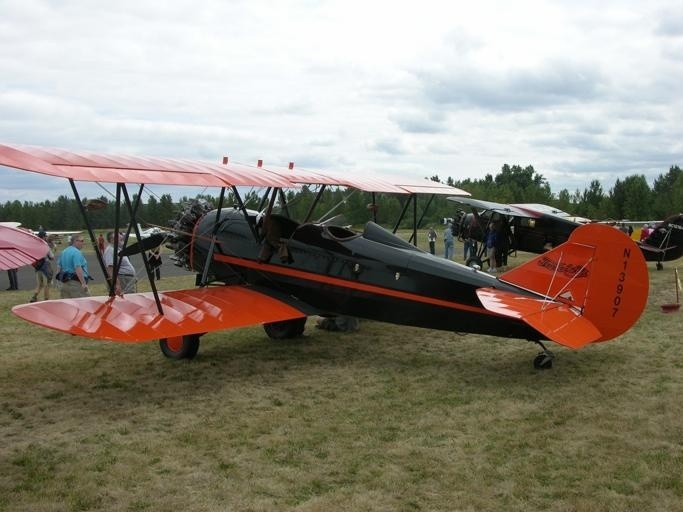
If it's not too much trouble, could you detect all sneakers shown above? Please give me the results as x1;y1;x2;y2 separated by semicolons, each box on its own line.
5;286;19;291
29;296;37;303
486;265;508;273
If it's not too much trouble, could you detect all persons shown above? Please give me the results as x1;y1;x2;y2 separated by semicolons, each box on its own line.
97;234;104;255
46;233;60;288
101;232;137;294
619;223;627;234
626;226;634;238
496;216;515;268
442;223;454;261
647;225;654;236
147;242;162;281
56;234;90;299
640;223;649;241
427;227;437;255
6;268;19;291
30;230;53;303
462;242;473;261
485;222;497;272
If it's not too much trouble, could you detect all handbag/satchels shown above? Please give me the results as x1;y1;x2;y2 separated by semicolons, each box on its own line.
55;271;73;282
33;258;45;270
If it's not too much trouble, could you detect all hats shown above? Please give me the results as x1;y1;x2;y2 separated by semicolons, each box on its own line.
38;231;46;237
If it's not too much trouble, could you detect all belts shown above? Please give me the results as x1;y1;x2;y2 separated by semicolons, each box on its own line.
119;273;134;277
